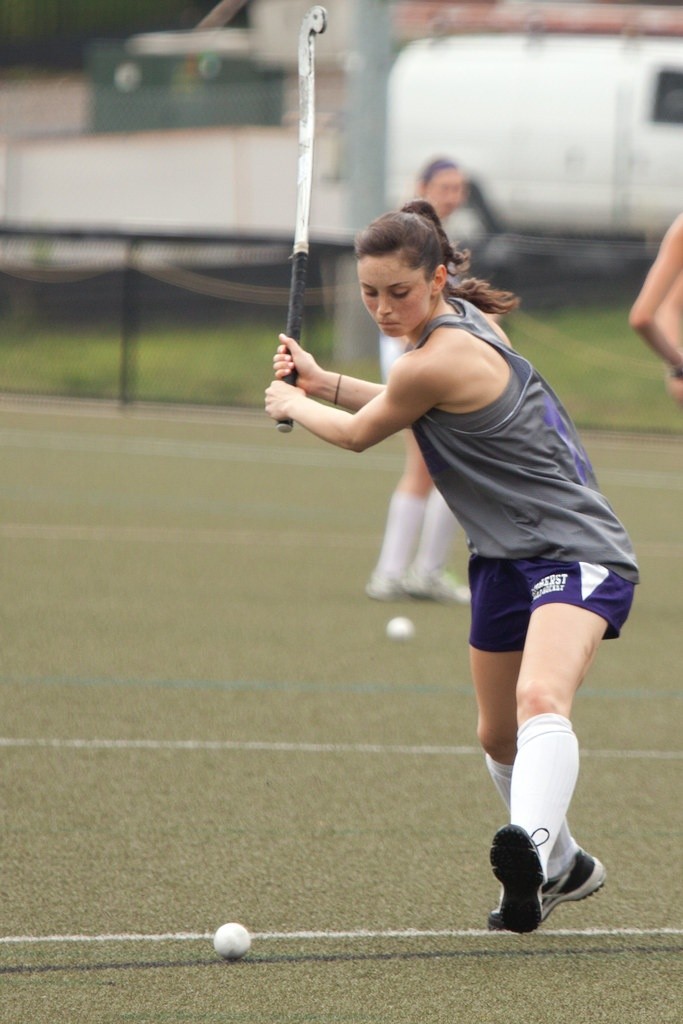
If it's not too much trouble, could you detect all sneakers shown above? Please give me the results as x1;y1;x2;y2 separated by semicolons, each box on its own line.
365;566;470;607
485;823;605;935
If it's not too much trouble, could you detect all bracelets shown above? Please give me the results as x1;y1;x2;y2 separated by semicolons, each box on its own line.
669;367;682;377
334;375;343;407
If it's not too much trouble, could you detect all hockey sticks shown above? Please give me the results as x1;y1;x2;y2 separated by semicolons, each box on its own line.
275;6;327;433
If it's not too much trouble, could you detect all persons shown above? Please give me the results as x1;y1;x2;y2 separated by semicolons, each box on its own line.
366;160;472;606
264;202;639;933
629;214;682;411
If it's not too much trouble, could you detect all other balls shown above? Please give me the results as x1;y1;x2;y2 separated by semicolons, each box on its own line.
212;922;253;961
385;616;417;647
